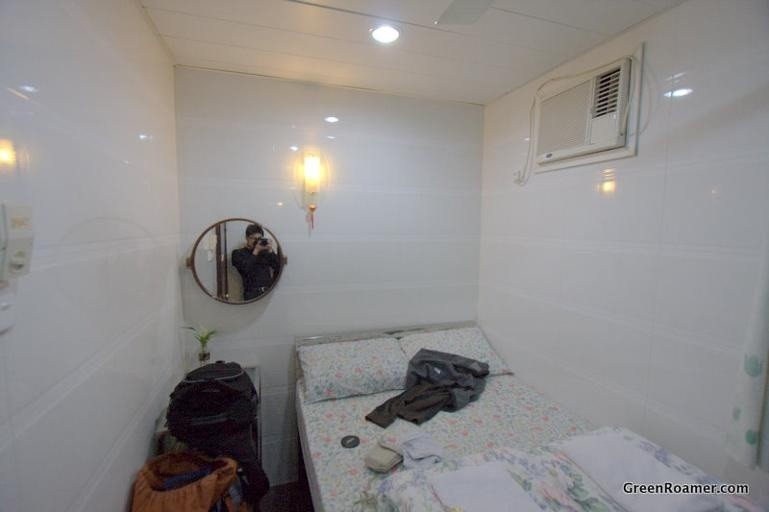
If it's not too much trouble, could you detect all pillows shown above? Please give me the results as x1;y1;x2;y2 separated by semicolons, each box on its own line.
394;328;513;376
295;338;409;404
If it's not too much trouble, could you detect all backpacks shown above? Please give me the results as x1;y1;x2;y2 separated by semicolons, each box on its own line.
165;360;269;502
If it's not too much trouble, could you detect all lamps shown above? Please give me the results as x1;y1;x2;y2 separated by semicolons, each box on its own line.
295;148;326;228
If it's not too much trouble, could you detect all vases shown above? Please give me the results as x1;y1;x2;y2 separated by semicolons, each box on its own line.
197;354;209;370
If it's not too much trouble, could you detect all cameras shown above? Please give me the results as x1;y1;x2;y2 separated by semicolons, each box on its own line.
254;237;268;247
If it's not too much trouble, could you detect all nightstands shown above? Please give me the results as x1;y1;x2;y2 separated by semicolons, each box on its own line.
157;366;262;465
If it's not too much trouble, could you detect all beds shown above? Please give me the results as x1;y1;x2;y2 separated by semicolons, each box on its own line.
295;321;769;511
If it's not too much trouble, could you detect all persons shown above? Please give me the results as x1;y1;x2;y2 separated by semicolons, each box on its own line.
232;224;280;300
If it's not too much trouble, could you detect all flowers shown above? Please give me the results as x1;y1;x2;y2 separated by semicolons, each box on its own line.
183;324;216;353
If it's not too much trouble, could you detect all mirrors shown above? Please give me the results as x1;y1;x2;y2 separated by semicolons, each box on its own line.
187;218;286;305
